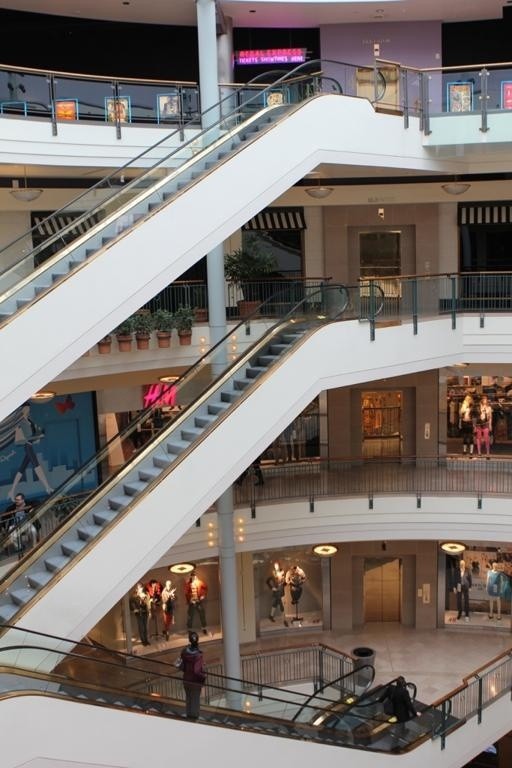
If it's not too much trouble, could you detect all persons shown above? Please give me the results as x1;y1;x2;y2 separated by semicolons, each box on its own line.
485;562;503;620
127;410;144;453
285;565;308;604
253;460;265;487
180;632;209;719
284;421;301;462
378;675;422;752
0;493;42;554
130;582;153;646
474;396;493;459
184;571;208;634
146;407;163;438
144;579;163;615
266;560;289;627
458;395;476;456
452;560;472;620
273;434;286;466
7;404;56;502
162;579;178;641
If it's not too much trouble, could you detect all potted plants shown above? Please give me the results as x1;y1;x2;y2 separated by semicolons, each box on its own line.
224;233;279;320
97;287;207;354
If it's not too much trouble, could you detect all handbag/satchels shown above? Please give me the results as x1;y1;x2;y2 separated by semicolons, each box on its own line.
15;427;26;444
384;701;396;716
175;658;184;669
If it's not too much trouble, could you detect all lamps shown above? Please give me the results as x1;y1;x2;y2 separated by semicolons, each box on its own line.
9;164;43;202
304;177;333;199
441;173;471;195
313;544;338;558
169;562;195;574
443;542;467;554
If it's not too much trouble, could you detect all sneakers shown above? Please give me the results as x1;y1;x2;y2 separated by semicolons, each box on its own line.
235;480;265;486
403;729;409;737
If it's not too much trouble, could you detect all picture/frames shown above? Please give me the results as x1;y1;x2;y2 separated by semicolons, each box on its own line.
51;89;290;124
0;390;102;512
446;80;512;113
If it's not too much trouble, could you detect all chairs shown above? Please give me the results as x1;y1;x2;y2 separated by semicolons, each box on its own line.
0;515;40;557
446;375;511;438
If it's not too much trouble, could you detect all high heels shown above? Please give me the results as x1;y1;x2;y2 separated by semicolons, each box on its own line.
47;488;54;494
7;493;16;501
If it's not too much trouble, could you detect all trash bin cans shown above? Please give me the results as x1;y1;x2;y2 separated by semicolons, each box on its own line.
352;647;376;687
